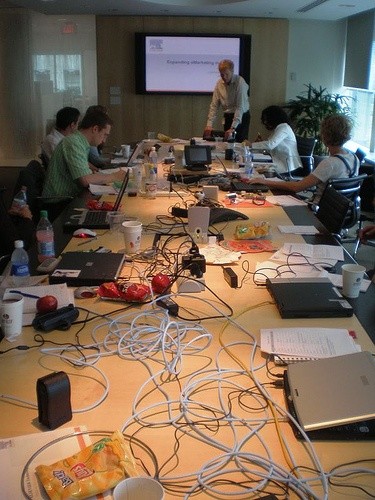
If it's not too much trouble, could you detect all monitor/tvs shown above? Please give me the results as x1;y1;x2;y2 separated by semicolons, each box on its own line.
184;145;212;166
133;32;251;97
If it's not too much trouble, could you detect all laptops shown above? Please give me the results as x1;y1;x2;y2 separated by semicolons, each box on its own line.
215;154;271;193
62;140;145;230
282;349;375;443
49;251;126;286
266;277;353;319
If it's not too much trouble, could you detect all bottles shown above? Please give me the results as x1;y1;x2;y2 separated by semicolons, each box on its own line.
11;239;33;286
36;210;55;264
128;147;161;200
11;186;27;210
224;133;255;176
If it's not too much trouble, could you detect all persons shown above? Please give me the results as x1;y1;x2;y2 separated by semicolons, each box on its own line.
246;112;361;216
77;104;116;171
41;106;102;176
204;59;252;143
357;224;375;245
41;103;126;222
241;104;305;177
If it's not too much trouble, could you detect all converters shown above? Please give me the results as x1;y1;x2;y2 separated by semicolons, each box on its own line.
224;268;238;288
156;295;178;315
33;303;80;331
171;207;188;218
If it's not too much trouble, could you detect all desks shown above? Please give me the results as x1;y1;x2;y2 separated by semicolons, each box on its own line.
0;140;375;500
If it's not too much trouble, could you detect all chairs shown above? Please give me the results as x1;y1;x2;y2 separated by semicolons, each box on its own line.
285;135;317;181
21;158;74;225
339;164;375;259
314;173;367;237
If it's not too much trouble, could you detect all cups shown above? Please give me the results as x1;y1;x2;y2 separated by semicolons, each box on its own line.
174;149;185;168
121;145;130;160
1;294;26;337
121;221;143;255
112;475;167;500
107;210;125;237
340;264;366;300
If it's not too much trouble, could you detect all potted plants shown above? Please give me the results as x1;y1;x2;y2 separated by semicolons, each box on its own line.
281;81;357;170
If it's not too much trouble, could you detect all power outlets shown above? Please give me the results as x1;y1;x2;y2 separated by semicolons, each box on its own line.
291;71;297;80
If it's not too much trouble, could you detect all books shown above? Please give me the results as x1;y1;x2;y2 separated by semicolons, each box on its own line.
264;194;309;207
273;343;362;366
1;282;72;314
250;152;273;163
86;183;117;197
0;286;74;328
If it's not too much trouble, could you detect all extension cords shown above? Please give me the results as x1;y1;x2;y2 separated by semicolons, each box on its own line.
173;254;205;293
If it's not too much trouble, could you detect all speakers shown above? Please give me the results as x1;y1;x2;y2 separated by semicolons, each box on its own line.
187;205;210;246
202;186;220;203
